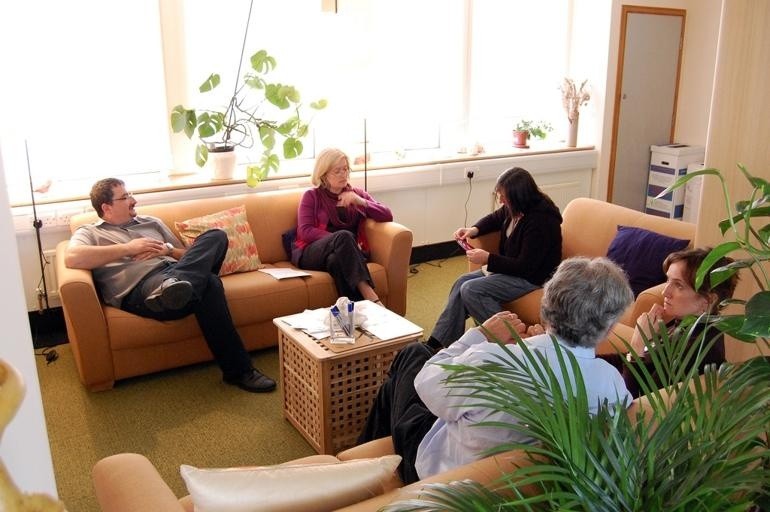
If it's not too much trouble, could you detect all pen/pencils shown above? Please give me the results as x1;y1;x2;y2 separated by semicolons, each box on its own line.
331;301;354;336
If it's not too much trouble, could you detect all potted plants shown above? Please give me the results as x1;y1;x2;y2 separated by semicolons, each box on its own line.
509;116;556;152
168;47;331;192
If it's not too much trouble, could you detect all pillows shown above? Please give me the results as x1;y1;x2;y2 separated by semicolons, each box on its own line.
170;204;265;280
601;222;691;305
175;454;398;510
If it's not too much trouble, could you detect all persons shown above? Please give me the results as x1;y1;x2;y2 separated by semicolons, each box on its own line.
596;246;739;402
292;147;394;313
63;177;278;393
354;254;636;489
424;165;564;353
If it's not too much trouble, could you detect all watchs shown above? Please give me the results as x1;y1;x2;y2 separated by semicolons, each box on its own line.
165;242;175;258
624;352;646;363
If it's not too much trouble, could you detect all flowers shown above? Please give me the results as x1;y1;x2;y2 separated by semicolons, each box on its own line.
555;74;595;119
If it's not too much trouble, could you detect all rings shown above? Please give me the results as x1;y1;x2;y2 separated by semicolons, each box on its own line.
508;314;514;320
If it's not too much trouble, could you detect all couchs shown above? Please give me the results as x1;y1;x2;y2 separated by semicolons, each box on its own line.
86;367;770;512
54;186;413;396
471;196;701;354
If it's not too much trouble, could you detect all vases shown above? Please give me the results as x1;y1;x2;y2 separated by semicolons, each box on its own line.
563;114;582;147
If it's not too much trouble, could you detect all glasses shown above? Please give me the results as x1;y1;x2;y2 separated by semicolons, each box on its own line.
111;192;132;201
456;238;474;251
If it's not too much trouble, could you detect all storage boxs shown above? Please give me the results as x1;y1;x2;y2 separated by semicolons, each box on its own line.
643;143;706;223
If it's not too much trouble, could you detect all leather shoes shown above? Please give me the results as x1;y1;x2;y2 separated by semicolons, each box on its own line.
144;277;193;313
223;366;276;392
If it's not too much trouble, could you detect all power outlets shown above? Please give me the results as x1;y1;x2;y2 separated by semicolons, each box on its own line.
464;168;476;183
